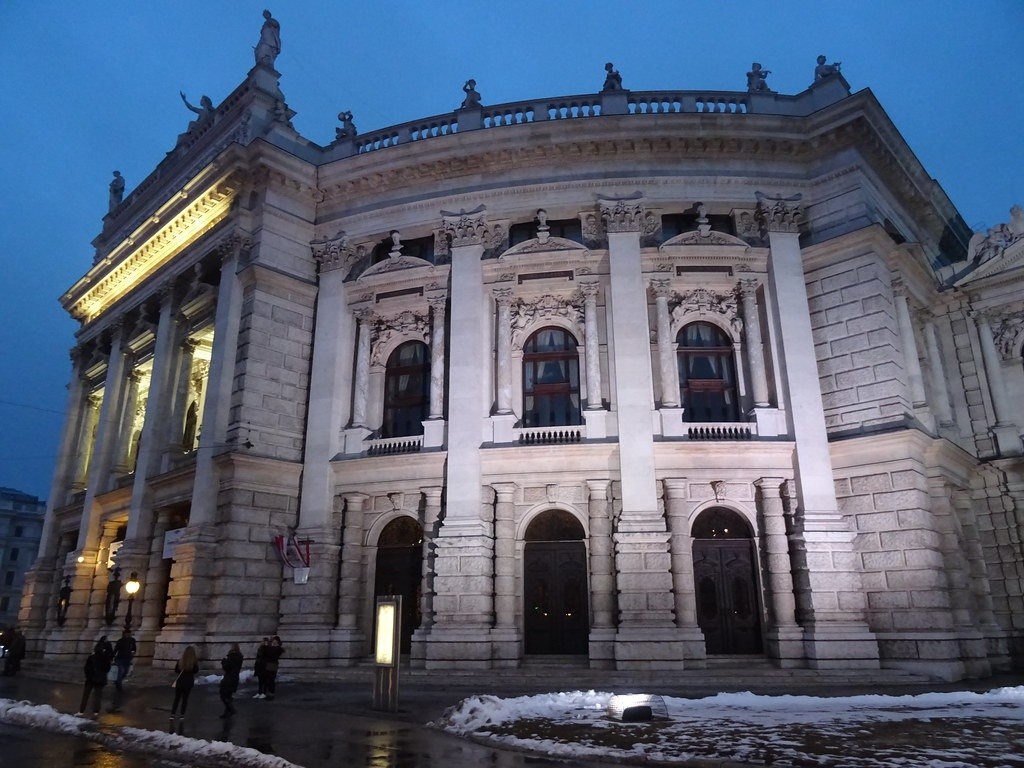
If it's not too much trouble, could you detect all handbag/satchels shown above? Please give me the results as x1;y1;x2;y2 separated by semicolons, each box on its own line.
171;680;176;687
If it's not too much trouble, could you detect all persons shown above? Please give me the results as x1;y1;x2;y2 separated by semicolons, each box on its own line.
109;170;126;212
603;62;622;90
220;643;244;718
747;63;770;90
815;55;841;80
461;79;483;108
255;9;282;70
253;635;283;699
166;90;215;155
644;212;661;247
371;321;433;358
74;628;137;716
510;305;584;346
583;215;601;249
490;225;507;258
436;232;450;264
391;233;404;250
336;110;357;139
537;211;550;229
974;230;1012;265
169;646;199;719
669;301;743;333
695;205;708;222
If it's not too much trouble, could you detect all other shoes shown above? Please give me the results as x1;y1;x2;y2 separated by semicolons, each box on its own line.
169;714;176;720
0;671;8;676
93;712;99;715
8;671;16;676
179;715;184;719
266;692;274;700
74;712;84;717
252;692;266;699
113;679;123;691
219;708;238;719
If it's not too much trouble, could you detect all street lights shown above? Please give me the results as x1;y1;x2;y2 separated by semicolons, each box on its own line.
123;570;142;631
